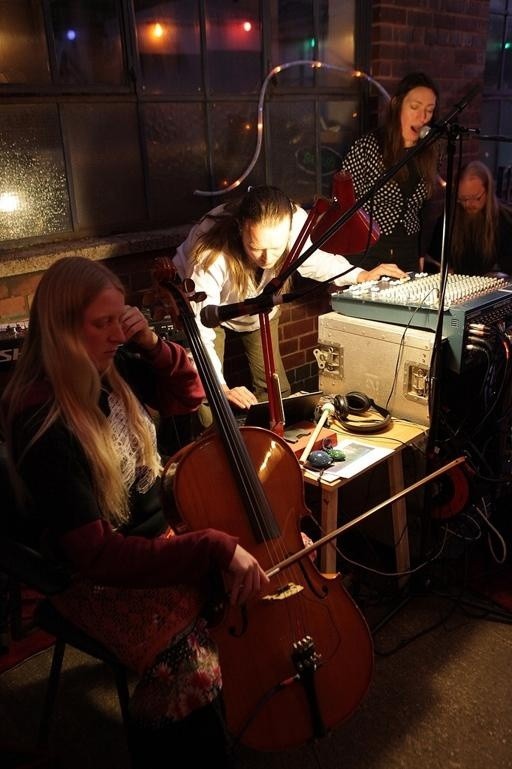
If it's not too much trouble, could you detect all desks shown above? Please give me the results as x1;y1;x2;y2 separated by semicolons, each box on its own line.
282;388;430;586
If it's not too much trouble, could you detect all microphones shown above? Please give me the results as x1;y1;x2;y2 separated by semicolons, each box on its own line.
421;126;460;144
199;289;294;329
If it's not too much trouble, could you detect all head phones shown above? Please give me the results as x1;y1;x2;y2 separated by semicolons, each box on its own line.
333;390;397;432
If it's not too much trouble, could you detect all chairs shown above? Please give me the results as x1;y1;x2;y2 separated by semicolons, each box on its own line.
0;442;224;767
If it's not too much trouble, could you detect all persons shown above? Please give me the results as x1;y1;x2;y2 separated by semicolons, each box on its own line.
5;256;276;762
170;185;407;428
339;69;442;272
442;159;510;279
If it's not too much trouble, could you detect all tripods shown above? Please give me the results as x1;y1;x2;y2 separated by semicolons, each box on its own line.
267;84;512;636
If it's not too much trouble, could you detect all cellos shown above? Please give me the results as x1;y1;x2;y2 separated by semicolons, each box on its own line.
154;254;375;752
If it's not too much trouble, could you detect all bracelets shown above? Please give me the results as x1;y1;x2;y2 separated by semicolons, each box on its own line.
141;341;161;356
